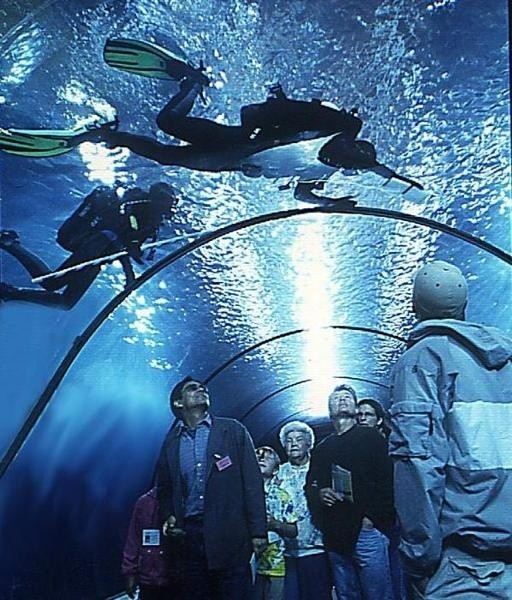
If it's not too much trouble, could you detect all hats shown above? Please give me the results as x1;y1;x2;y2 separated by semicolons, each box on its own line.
411;259;470;317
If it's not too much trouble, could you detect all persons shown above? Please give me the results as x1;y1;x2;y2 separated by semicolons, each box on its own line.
0;182;177;311
123;374;411;599
387;261;512;599
59;61;395;206
